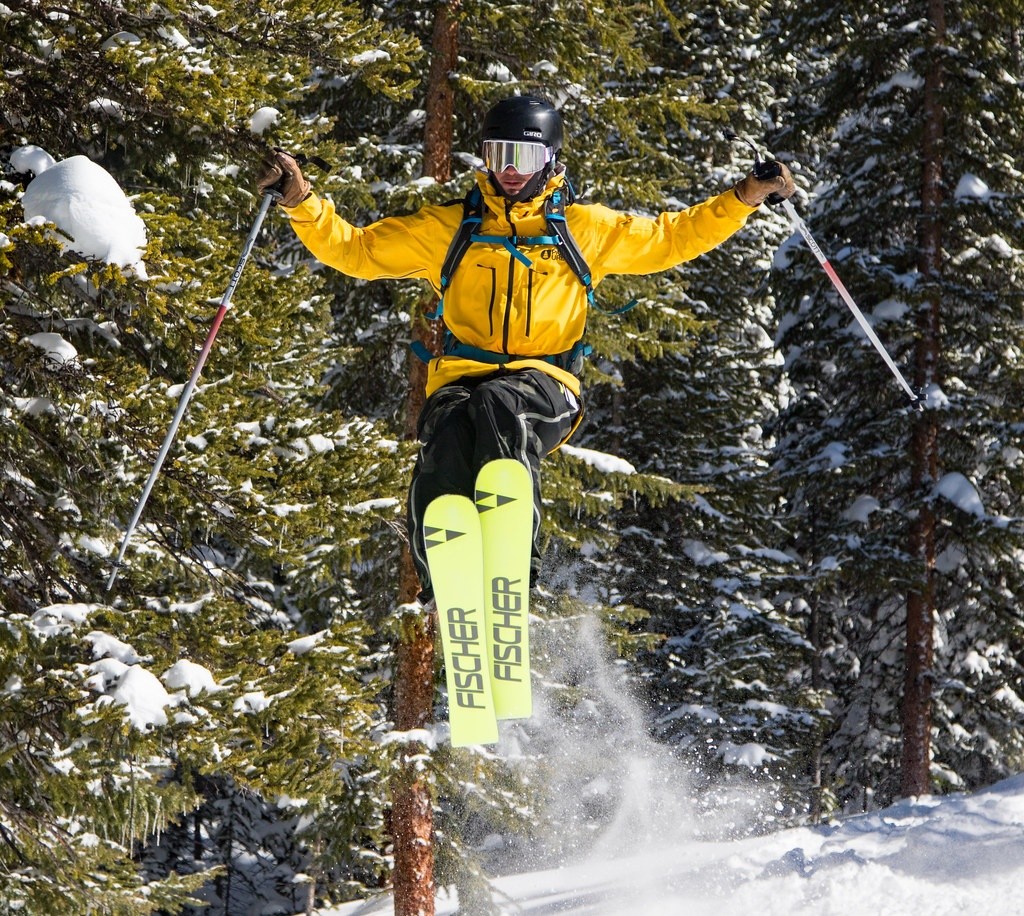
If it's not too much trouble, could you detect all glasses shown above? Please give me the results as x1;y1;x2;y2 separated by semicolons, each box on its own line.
481;139;547;175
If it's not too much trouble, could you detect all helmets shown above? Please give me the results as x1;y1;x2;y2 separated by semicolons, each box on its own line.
481;96;564;179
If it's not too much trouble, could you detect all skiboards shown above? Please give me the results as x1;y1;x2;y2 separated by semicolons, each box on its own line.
422;458;535;748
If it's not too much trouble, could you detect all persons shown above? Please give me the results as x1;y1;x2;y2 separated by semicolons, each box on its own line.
258;93;798;608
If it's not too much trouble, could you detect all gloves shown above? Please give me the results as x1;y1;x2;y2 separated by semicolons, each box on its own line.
257;146;311;208
735;161;796;209
